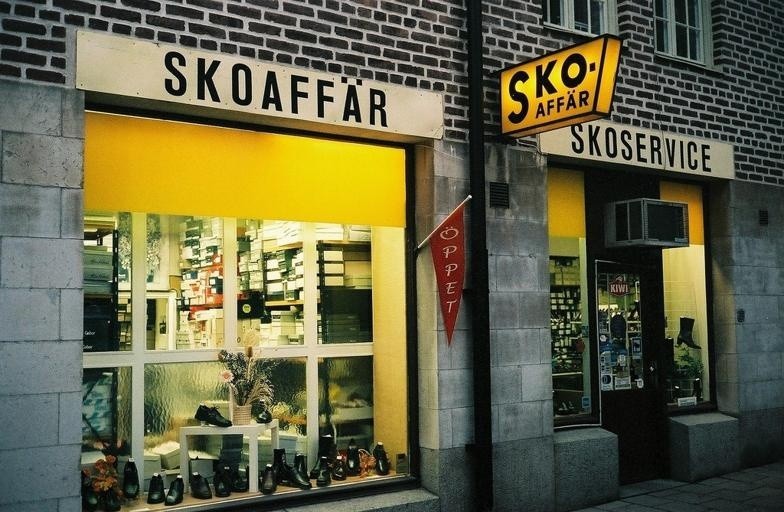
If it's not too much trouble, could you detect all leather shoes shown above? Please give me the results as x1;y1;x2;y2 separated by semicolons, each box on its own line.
254;408;272;424
188;470;212;501
260;463;276;493
344;438;362;476
212;462;247;498
371;440;389;476
315;455;346;486
121;460;141;500
146;472;165;504
275;462;312;492
556;400;580;416
194;404;232;427
164;474;185;506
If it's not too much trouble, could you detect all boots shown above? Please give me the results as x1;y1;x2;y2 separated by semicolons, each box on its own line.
676;316;704;350
272;447;287;483
293;450;310;482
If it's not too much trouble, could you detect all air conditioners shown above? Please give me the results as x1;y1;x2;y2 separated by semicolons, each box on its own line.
601;195;690;255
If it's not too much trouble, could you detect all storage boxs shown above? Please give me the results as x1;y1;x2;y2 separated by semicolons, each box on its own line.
78;432;304;496
173;214;368;350
